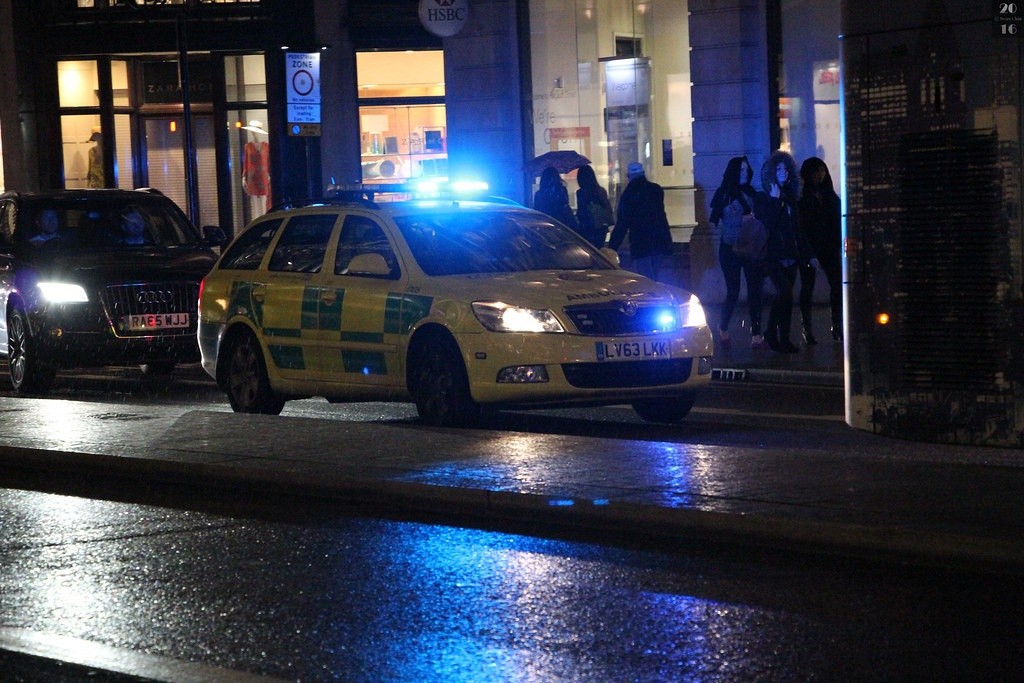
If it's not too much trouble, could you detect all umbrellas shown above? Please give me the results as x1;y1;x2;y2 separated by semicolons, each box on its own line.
520;150;590;178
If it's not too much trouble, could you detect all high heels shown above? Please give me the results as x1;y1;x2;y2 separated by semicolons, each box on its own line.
831;322;843;341
801;324;817;344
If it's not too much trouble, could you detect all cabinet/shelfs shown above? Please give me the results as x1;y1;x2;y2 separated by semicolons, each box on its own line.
362;152;446;196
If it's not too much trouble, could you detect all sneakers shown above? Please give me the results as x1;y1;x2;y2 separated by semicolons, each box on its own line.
715;323;730;341
749;335;763;347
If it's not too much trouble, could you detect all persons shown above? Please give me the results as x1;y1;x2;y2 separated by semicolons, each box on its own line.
754;151;822;354
85;132;105;189
607;162;675;281
797;157;842;344
709;155;766;351
240;123;271;222
532;167;578;233
576;164;610;249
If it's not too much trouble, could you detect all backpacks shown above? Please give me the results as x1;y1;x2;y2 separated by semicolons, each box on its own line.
586;198;615;230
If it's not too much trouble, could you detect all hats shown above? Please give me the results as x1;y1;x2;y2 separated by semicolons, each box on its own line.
625;162;645;174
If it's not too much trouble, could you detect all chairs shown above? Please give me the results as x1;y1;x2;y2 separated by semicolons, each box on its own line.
359;230;392;264
77;211;117;249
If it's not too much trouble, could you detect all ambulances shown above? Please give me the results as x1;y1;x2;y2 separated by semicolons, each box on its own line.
196;179;713;430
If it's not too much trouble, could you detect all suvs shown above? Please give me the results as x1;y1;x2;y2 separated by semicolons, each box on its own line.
0;186;230;395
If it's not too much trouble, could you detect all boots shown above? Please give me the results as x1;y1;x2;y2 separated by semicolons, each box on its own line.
763;319;780;352
781;328;799;355
